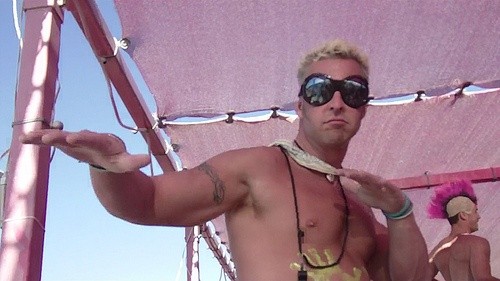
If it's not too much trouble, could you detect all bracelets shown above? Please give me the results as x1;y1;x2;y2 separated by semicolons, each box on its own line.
381;192;415;219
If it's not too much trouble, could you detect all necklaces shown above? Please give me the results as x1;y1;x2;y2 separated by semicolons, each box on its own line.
279;139;350;281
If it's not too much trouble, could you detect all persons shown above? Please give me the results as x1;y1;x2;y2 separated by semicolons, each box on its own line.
18;39;430;281
426;177;500;281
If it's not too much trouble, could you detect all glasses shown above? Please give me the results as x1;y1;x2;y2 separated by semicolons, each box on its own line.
298;73;374;108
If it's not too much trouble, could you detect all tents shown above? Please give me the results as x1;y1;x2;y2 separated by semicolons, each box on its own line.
0;0;500;281
